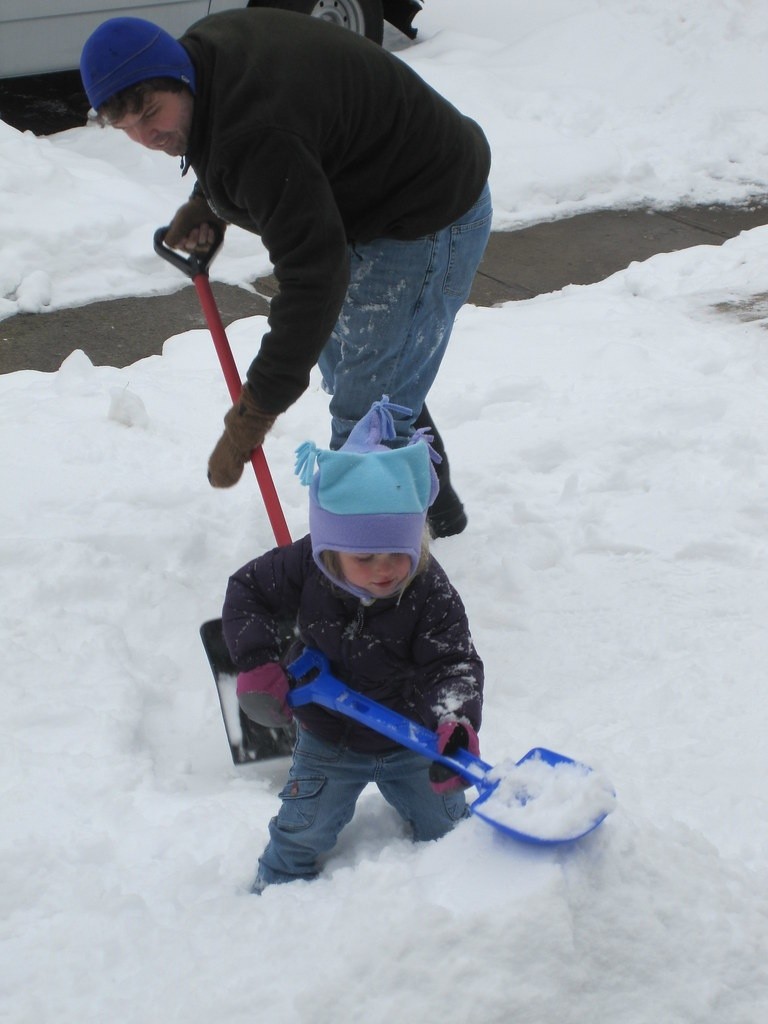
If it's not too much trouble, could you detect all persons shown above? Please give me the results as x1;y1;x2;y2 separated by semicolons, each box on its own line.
79;2;501;547
220;390;491;895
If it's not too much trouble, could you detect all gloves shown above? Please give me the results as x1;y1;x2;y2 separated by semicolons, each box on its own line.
207;386;279;490
235;661;292;729
428;714;480;795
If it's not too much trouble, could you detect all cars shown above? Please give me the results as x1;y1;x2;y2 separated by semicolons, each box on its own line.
0;0;384;82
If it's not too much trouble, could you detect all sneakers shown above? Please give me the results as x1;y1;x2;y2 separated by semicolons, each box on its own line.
428;484;468;538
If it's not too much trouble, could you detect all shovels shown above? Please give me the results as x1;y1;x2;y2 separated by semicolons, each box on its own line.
155;227;294;768
280;645;618;845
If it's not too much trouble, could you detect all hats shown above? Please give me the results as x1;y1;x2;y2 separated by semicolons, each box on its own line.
293;397;440;605
79;17;196;110
